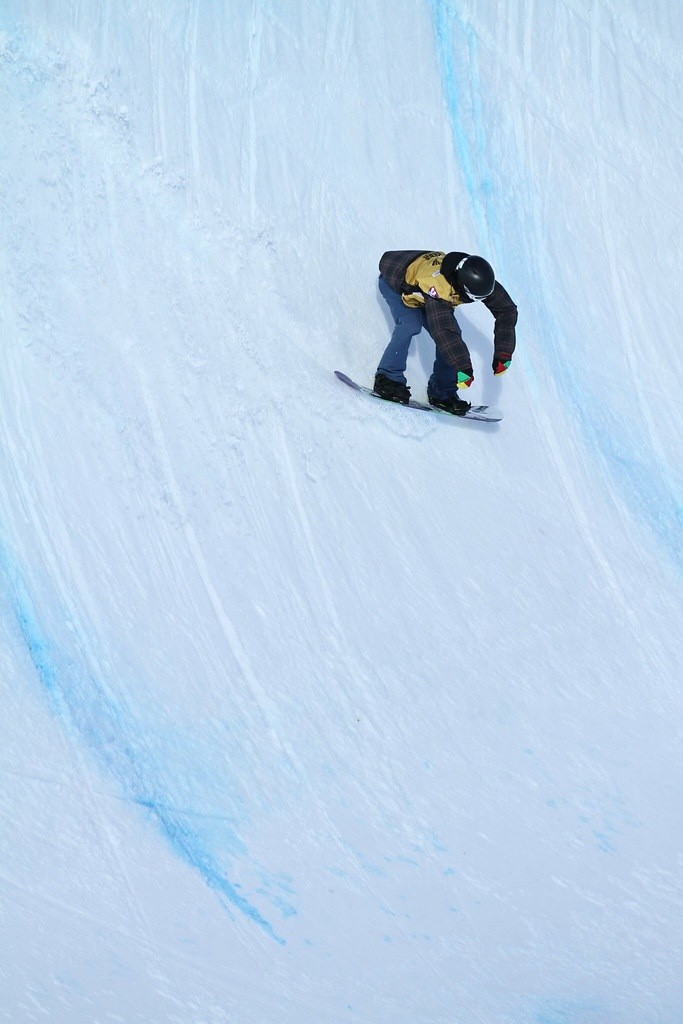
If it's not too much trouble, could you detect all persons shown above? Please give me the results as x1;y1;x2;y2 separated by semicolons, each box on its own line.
378;250;519;415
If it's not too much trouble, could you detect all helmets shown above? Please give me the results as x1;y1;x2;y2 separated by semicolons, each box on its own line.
459;256;494;296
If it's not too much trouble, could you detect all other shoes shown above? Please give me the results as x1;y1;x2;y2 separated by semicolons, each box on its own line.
428;391;470;412
374;373;411;403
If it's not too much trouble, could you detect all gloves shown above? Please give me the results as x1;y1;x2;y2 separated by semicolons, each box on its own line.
492;352;513;376
457;369;474;389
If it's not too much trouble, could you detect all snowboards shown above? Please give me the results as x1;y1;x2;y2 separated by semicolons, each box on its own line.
332;370;501;424
